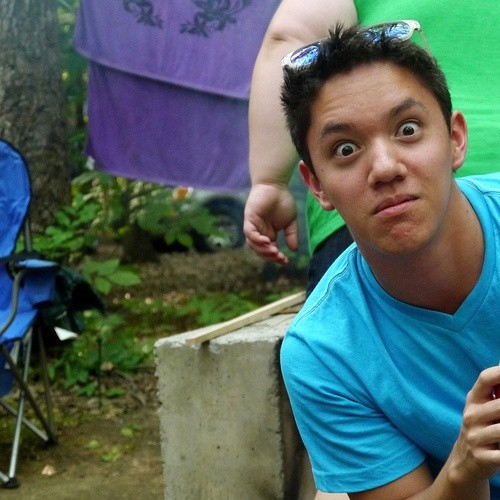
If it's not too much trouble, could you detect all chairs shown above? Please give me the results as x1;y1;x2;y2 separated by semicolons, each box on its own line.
1;138;62;496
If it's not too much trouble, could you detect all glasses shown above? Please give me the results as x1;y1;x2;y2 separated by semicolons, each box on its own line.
280;19;433;73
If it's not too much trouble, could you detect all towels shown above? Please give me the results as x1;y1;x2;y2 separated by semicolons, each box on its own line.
71;0;282;202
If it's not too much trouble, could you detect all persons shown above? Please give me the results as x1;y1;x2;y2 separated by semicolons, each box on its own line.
243;0;500;301
278;19;500;500
